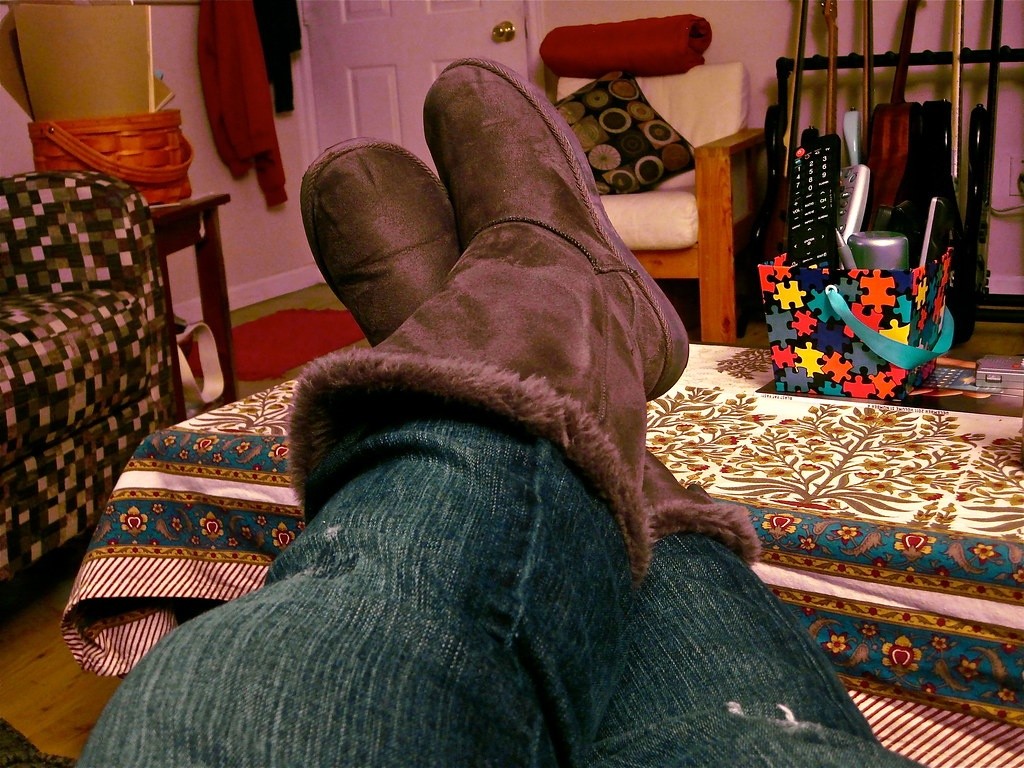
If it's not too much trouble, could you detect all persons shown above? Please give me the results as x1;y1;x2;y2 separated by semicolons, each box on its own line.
75;56;934;768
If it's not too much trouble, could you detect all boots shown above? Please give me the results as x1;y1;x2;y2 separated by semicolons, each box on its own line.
300;137;761;568
287;57;690;584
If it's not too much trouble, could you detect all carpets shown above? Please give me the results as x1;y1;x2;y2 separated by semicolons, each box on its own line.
229;307;366;383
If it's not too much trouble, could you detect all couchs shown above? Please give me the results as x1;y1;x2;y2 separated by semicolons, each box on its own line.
553;59;781;341
0;171;177;581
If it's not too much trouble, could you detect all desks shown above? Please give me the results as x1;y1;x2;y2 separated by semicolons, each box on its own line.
153;193;237;423
59;341;1024;768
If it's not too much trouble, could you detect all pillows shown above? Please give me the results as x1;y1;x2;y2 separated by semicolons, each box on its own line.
554;70;696;195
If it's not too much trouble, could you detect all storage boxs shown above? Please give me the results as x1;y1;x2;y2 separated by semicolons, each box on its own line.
756;239;954;401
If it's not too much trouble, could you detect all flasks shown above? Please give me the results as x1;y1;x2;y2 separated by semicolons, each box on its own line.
848;230;909;271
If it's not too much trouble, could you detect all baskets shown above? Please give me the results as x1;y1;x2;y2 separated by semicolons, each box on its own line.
27;109;193;205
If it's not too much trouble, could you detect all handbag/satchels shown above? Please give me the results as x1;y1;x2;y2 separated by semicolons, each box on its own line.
757;227;953;403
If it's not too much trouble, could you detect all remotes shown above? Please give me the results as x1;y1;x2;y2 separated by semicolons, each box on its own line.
787;133;949;271
